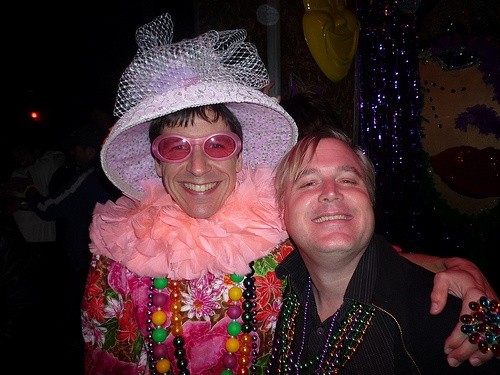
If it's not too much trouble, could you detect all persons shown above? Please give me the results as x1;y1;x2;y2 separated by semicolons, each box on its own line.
260;81;341;140
250;126;500;375
16;127;122;296
82;13;500;375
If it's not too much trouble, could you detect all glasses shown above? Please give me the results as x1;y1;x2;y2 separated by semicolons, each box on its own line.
151;133;242;163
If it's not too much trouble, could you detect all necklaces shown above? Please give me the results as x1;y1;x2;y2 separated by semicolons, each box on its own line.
265;275;377;375
147;260;259;375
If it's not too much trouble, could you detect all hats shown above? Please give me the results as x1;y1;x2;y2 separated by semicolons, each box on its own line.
100;13;300;201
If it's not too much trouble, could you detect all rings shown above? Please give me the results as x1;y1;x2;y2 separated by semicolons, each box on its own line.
460;295;500;355
